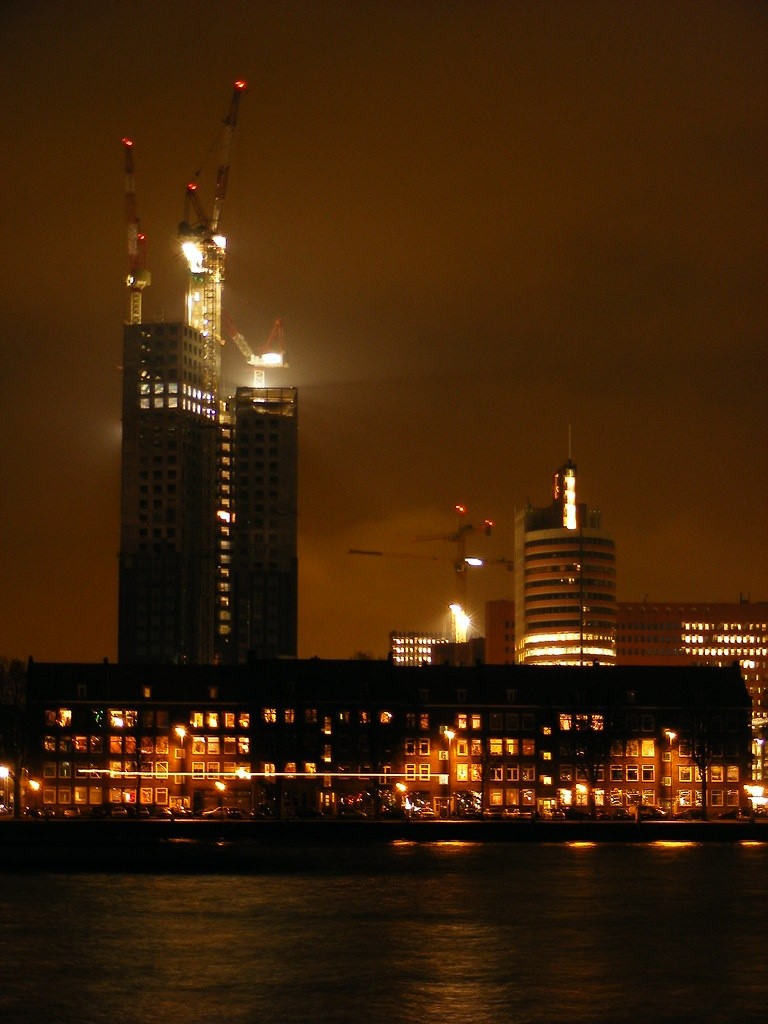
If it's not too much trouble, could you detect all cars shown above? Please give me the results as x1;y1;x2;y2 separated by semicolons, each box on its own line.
292;805;324;820
737;806;755;823
672;808;702;819
225;806;243;819
202;805;234;819
337;807;368;820
378;804;671;820
0;802;15;816
250;808;265;819
717;808;738;819
21;801;193;819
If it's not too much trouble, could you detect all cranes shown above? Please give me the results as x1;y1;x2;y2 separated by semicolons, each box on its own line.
348;502;514;647
118;73;288;398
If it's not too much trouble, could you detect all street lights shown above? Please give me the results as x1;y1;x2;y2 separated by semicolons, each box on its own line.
215;781;225;820
443;732;455;819
174;727;186;794
577;784;586;807
669;733;678;818
